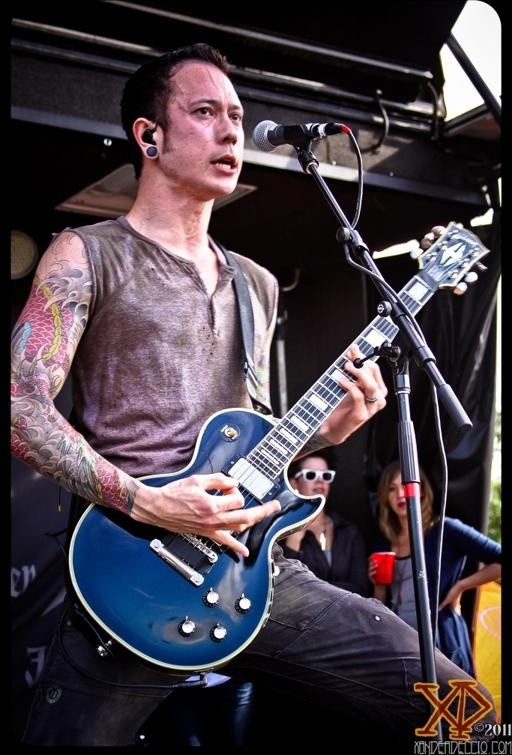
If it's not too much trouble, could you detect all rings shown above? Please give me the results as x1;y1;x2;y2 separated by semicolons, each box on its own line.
365;396;378;402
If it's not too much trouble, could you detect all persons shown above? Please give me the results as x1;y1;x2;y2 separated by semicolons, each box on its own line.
275;452;371;599
369;460;502;679
10;42;494;755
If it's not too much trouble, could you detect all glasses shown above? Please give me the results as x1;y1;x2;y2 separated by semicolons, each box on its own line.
295;469;336;483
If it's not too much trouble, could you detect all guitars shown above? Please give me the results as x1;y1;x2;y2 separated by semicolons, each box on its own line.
69;221;492;673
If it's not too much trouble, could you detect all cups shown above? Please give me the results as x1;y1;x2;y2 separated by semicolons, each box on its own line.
369;551;397;586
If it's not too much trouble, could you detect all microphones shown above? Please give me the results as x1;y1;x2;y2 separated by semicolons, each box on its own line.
251;120;343;152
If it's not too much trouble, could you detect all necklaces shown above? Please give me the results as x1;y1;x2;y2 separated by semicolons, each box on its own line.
316;511;328;551
393;539;412;607
